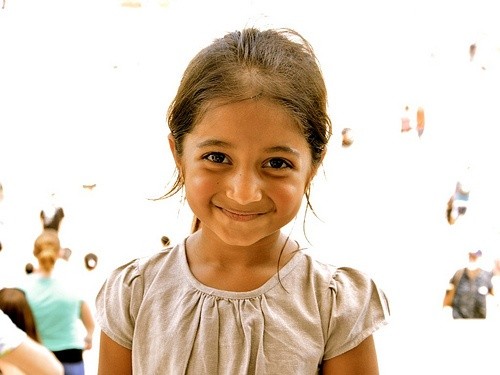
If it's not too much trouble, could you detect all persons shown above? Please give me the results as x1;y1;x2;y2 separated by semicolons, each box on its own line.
443;251;494;319
1;230;94;375
93;26;393;375
